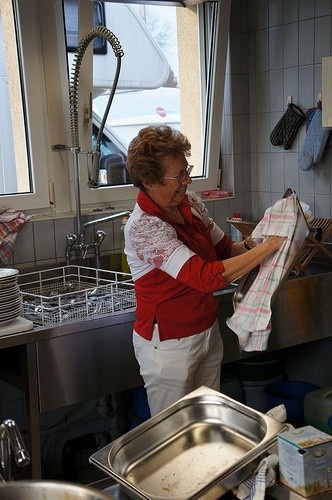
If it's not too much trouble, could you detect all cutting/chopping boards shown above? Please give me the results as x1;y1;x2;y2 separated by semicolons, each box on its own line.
0;317;34;336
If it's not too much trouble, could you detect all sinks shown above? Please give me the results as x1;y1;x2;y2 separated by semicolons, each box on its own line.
18;279;144;416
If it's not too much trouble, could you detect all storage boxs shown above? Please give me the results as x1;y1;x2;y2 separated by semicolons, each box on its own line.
276;425;332;499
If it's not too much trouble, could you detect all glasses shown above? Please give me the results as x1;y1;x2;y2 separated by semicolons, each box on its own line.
160;164;194;183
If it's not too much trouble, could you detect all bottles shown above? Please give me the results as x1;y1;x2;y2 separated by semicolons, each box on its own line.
119;216;132;278
230;213;243;242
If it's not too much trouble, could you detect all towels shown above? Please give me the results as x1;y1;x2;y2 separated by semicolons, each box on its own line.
225;192;310;353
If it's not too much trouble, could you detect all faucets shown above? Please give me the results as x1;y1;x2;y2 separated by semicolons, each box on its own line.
65;233;78;259
0;419;30;482
94;229;107;256
80;211;131;251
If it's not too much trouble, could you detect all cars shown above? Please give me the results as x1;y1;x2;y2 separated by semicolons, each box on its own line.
88;109;132;187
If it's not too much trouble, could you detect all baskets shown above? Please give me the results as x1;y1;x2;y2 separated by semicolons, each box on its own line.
17;264;138;327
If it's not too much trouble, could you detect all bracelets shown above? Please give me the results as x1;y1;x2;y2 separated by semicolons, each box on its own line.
244;241;250;251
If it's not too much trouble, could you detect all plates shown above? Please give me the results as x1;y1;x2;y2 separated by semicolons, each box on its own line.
0;267;22;326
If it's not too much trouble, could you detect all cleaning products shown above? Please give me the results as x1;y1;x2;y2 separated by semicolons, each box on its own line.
121;215;131;280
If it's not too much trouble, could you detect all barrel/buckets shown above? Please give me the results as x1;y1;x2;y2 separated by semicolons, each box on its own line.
129;385;151;431
219;372;283;413
261;380;332;436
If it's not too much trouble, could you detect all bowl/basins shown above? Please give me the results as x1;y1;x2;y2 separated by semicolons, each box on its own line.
225;355;279;380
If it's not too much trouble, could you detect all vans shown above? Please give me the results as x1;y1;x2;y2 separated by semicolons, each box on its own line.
61;0;182;149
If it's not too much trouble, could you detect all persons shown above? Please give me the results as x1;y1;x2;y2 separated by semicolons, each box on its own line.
125;125;286;422
